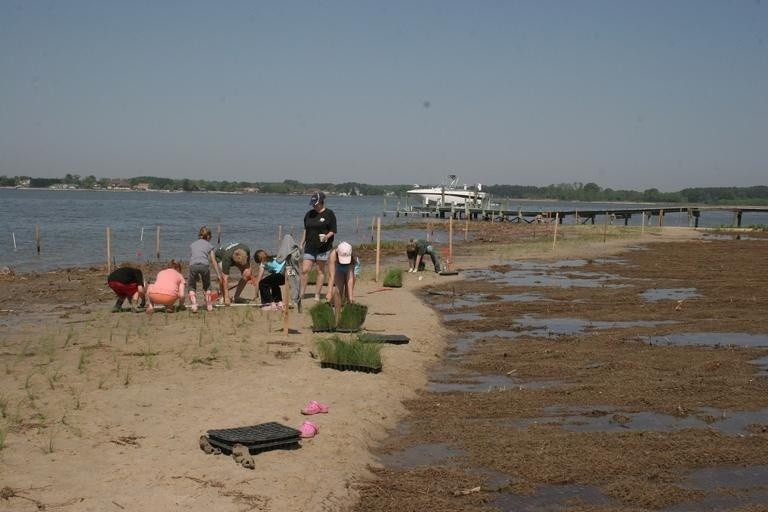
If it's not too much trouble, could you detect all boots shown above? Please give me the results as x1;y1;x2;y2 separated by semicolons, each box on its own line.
131;298;145;314
111;299;124;313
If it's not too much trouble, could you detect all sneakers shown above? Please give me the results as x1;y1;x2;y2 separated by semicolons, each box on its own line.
146;307;154;313
166;305;174;312
191;308;198;312
261;303;284;312
207;305;212;311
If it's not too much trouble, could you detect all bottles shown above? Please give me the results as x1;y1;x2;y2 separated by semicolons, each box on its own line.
354;257;360;278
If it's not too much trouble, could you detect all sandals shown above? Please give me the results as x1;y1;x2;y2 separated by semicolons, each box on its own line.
301;400;328;415
199;435;222;455
299;421;320;437
232;443;255;468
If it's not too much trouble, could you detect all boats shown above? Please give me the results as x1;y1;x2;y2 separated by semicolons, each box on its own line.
406;176;486;211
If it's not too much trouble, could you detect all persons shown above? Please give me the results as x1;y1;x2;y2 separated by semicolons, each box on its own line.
406;239;441;273
186;225;222;313
300;192;338;302
108;267;145;313
145;259;185;313
253;250;287;311
326;242;360;322
215;242;256;306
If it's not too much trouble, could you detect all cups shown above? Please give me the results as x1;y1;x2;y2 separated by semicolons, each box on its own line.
318;233;326;242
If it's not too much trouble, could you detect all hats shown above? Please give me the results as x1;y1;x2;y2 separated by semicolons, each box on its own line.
309;192;321;205
407;239;416;251
338;241;353;265
